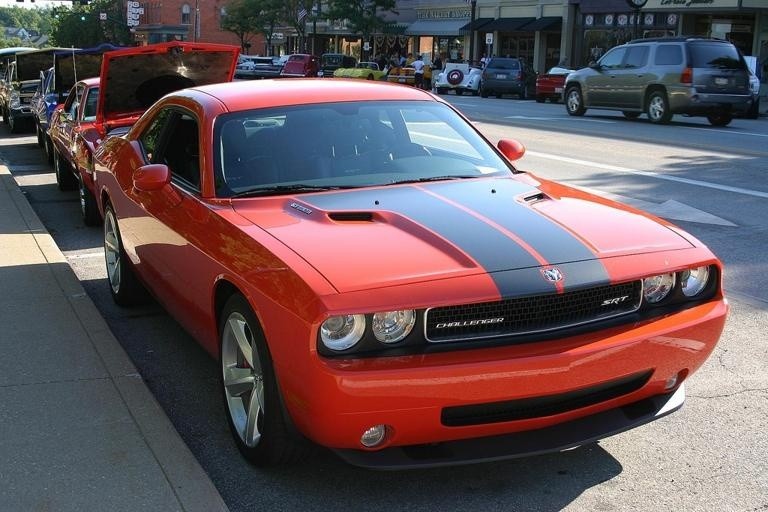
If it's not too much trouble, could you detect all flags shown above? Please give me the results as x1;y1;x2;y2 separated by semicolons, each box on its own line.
296;2;308;23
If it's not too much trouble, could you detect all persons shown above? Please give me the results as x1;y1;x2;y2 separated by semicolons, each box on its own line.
369;53;493;89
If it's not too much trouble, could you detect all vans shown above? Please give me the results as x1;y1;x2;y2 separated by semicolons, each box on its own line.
481;54;533;100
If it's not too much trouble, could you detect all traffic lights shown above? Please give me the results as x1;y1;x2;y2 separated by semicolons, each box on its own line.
55;7;59;19
81;8;85;21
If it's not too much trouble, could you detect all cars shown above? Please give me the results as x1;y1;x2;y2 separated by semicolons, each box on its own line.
742;55;760;119
535;64;587;103
0;47;40;79
435;59;485;96
232;51;388;83
387;64;432;91
29;43;133;165
0;47;84;135
46;41;242;228
92;78;729;472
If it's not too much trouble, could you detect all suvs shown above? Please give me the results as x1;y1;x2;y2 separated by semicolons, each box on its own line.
561;37;753;127
